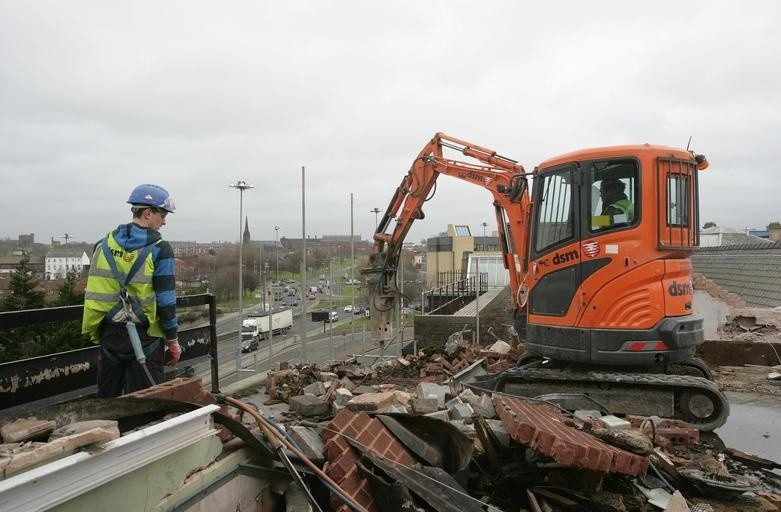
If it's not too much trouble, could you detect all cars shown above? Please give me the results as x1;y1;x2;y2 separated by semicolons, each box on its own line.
240;273;370;353
403;304;422;314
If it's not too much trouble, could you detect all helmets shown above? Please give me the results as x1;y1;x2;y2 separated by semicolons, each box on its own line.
127;183;176;214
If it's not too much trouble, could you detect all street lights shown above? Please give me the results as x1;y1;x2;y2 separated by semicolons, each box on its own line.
61;233;72;279
369;207;383;231
229;180;255;369
272;225;280;281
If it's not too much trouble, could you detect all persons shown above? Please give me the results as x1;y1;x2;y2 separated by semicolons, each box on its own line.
81;184;183;398
598;177;634;230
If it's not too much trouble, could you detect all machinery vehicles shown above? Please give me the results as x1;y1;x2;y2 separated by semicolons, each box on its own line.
361;132;731;432
